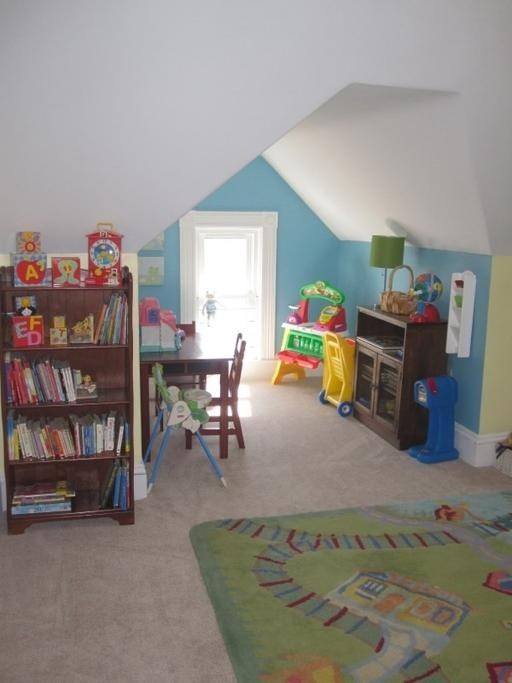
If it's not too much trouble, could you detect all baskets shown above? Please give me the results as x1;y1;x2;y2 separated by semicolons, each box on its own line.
379;265;418;316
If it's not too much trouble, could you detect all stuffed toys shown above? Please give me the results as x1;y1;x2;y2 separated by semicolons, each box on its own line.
202;290;226;327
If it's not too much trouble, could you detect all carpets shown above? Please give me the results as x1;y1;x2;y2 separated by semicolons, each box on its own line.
190;492;512;682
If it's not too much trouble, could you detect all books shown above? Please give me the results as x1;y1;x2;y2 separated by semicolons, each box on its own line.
2;290;131;518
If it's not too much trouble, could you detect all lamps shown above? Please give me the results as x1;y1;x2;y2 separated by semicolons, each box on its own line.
368;236;404;309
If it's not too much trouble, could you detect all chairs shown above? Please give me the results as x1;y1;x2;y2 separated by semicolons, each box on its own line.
154;322;246;450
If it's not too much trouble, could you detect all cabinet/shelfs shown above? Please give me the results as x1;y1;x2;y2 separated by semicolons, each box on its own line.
350;304;448;450
0;267;134;535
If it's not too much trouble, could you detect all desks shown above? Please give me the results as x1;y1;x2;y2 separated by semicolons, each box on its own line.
140;333;234;462
